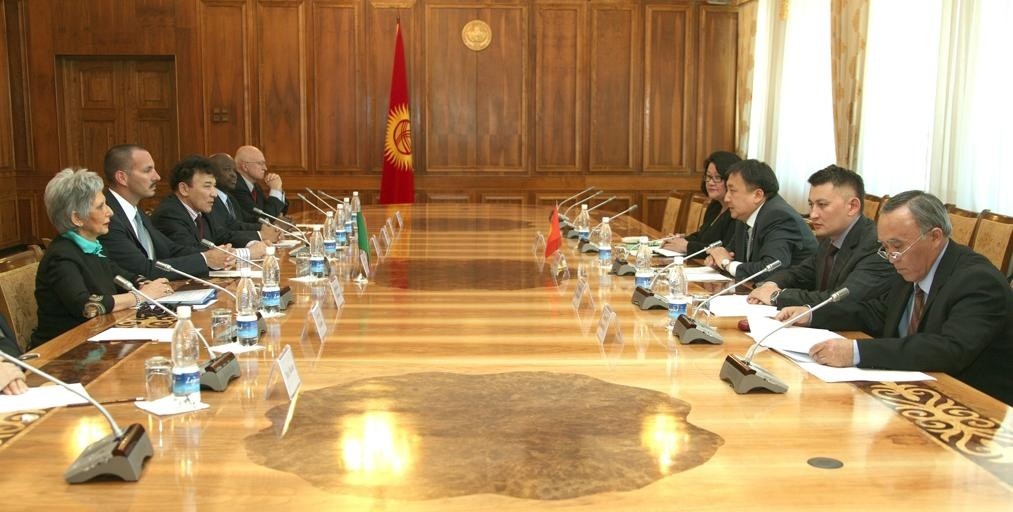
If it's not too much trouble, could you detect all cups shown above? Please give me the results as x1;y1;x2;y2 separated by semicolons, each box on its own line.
145;356;172;399
617;245;628;261
691;294;709;326
295;253;309;275
592;227;601;240
654;268;669;285
211;308;232;344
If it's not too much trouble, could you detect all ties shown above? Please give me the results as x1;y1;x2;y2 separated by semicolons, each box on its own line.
905;282;924;337
250;187;256;203
194;214;205;240
225;195;235;220
134;210;150;260
819;244;838;292
743;223;750;263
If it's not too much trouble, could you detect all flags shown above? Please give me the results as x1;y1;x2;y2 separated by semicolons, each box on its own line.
357;207;370;268
545;207;562;259
377;15;415;205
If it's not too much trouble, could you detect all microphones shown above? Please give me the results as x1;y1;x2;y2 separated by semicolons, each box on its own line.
306;187;337;212
257;216;331;277
113;274;241;392
578;204;638;253
719;287;850;394
563;195;617;239
611;233;686;276
253;207;310;256
558;185;595;222
672;259;782;344
559;190;604;230
296;192;327;215
0;350;154;484
631;239;723;310
200;238;295;310
155;260;267;342
318;189;344;204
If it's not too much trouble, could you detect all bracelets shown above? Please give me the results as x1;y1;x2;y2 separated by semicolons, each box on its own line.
129;291;141;307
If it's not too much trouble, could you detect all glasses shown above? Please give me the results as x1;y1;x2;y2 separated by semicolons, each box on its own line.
243;161;265;167
702;174;725;183
877;229;934;261
135;301;181;319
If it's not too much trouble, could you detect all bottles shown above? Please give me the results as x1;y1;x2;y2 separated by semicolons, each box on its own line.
578;204;590;240
323;212;336;258
351;191;360;211
171;306;199;396
598;217;611;259
334;204;346;246
343;198;354;236
668;256;688;328
309;226;324;277
635;236;654;287
237;267;258;345
261;247;279;313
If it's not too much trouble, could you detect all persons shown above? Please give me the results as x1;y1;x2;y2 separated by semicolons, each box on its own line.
150;155;273;261
231;145;290;225
703;158;817;283
96;144;237;281
32;167;174;350
775;191;1013;405
747;164;900;311
208;153;283;248
0;314;28;396
660;150;745;260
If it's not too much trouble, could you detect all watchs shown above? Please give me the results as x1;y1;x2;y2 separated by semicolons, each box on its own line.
721;258;734;271
770;289;783;306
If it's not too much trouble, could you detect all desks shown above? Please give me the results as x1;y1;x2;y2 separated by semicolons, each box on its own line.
0;200;1013;512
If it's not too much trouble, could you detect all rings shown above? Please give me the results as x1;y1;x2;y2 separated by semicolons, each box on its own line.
167;287;170;294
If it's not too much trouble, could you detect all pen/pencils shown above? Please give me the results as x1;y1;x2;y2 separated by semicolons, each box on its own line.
66;396;144;407
95;339;157;343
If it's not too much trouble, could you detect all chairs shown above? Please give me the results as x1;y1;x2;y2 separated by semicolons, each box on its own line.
684;196;702;237
964;209;1013;276
696;203;708;234
0;243;45;352
862;193;884;223
661;194;683;238
939;206;978;251
